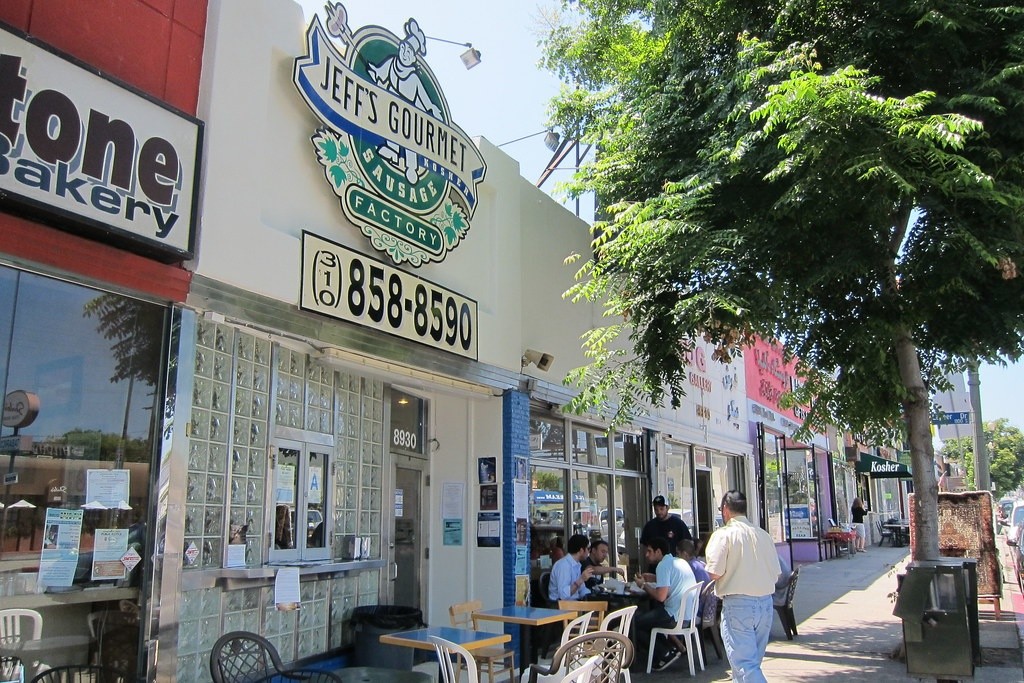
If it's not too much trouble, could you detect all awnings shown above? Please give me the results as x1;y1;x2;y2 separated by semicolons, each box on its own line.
856;451;912;479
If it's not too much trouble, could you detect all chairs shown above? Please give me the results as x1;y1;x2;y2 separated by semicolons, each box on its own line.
0;607;143;683
209;565;803;683
876;518;911;547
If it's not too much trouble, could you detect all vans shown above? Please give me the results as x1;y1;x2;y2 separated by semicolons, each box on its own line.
667;509;719;532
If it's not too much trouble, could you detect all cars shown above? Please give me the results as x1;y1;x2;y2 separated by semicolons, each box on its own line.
999;500;1024;595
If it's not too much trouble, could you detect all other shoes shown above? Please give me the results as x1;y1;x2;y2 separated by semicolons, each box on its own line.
652;646;682;671
858;549;866;552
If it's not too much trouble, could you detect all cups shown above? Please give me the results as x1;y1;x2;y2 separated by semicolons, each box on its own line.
615;582;624;595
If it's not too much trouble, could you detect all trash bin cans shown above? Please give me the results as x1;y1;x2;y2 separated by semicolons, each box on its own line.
896;556;981;676
345;605;422;671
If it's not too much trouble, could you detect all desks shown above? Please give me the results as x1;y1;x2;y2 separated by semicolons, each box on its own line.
470;605;578;683
379;625;511;683
822;531;857;559
882;524;909;545
0;635;97;683
582;579;674;672
299;666;433;683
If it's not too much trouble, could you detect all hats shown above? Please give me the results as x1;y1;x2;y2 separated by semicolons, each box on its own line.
652;494;668;506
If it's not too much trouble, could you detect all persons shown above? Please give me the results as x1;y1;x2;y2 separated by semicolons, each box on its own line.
639;496;693;574
580;541;624;592
704;490;782;683
677;532;791;629
851;498;868;552
548;534;595;620
634;531;699;671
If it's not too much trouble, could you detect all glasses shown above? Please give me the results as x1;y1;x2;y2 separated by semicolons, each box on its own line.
718;506;724;511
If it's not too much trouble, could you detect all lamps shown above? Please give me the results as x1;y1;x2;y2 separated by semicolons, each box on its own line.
424;35;482;70
495;127;561;153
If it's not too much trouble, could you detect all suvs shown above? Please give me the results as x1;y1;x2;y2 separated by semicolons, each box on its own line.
289;510;323;547
573;508;625;543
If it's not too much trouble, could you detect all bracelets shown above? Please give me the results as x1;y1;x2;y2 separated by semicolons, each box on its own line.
574;581;578;589
641;583;647;590
